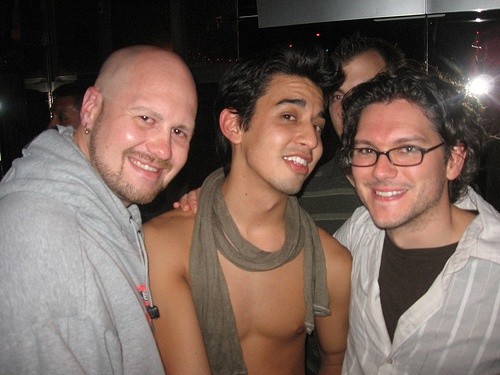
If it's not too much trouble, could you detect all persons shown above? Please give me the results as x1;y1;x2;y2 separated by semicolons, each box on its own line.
0;44;198;375
173;39;500;375
143;43;352;375
47;81;190;222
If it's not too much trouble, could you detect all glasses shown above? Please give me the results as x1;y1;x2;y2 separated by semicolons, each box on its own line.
347;142;443;167
